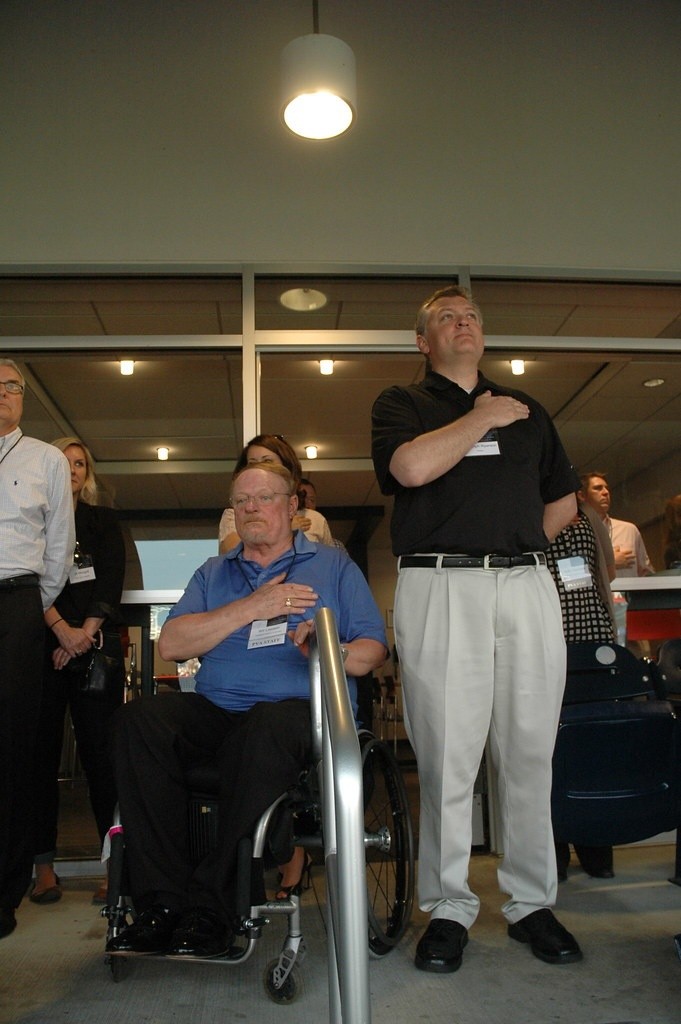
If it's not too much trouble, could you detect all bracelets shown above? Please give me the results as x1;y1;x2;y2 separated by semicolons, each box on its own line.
50;618;63;630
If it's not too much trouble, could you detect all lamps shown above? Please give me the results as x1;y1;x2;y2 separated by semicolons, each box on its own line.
318;359;334;376
305;444;318;460
274;0;360;141
119;359;136;375
510;359;525;375
155;445;169;460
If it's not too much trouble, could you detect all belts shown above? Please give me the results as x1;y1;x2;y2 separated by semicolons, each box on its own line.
0;576;37;589
399;554;546;570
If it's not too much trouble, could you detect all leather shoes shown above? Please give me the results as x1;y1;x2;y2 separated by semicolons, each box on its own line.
415;918;468;973
107;906;168;951
167;906;236;958
508;908;584;964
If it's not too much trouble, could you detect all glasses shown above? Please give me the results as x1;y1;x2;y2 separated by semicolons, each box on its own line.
274;434;285;443
1;382;24;394
230;490;290;507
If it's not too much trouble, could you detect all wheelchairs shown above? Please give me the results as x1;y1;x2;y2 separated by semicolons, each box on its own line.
101;635;415;1005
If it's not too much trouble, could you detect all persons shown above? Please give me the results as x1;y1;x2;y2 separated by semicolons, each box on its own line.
663;495;681;569
0;357;76;938
31;438;126;904
106;466;389;958
546;508;617;882
578;472;654;650
369;286;583;973
219;434;348;557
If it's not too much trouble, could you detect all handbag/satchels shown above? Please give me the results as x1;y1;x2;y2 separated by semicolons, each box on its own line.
59;628;107;692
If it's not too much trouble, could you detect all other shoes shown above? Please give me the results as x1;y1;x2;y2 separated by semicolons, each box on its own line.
30;873;62;903
93;886;107;902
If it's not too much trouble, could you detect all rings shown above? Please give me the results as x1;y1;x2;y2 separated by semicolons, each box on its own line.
77;649;82;653
285;598;292;607
